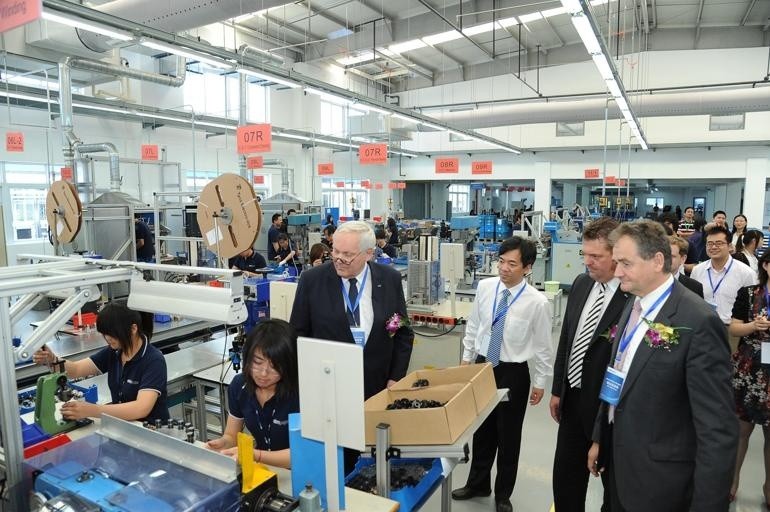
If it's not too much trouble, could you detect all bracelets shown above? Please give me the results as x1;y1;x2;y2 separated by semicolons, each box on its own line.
256;449;261;463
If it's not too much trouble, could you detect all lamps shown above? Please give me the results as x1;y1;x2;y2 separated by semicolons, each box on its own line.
41;0;649;159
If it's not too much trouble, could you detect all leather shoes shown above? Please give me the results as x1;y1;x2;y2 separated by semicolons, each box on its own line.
452;487;491;500
495;497;512;512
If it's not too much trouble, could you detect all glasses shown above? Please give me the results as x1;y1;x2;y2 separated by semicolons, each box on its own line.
332;252;360;264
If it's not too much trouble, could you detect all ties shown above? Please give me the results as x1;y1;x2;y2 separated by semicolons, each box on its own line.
486;289;511;368
567;283;607;388
347;278;360;326
608;301;641;423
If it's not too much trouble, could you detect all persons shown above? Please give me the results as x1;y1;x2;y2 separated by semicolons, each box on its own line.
549;217;634;512
290;220;414;477
470;204;532;225
452;236;554;512
588;218;740;512
135;214;155;262
232;209;398;279
33;298;170;426
205;317;300;469
645;205;770;360
729;249;770;512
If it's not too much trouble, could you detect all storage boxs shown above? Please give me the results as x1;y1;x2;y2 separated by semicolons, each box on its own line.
364;381;479;445
388;362;497;414
